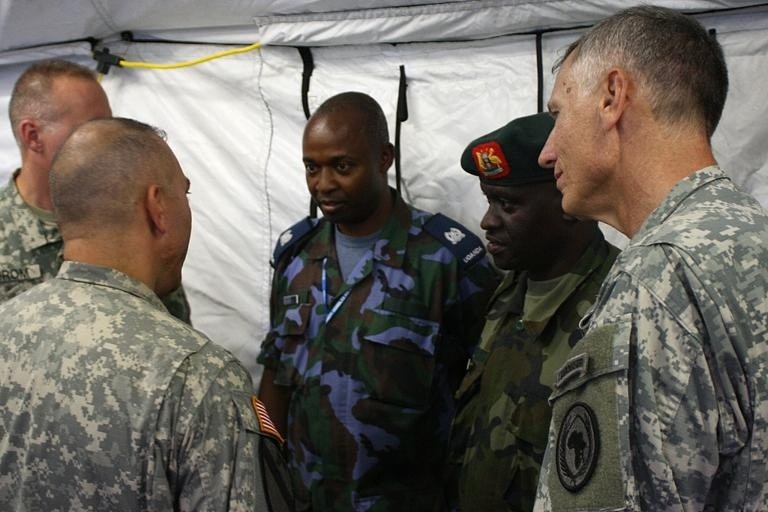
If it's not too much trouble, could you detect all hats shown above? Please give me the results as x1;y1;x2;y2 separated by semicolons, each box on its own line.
459;111;561;183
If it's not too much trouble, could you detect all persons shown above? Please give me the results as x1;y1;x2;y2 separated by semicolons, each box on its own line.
439;111;620;512
531;5;764;512
257;91;507;511
0;117;295;512
0;56;191;324
